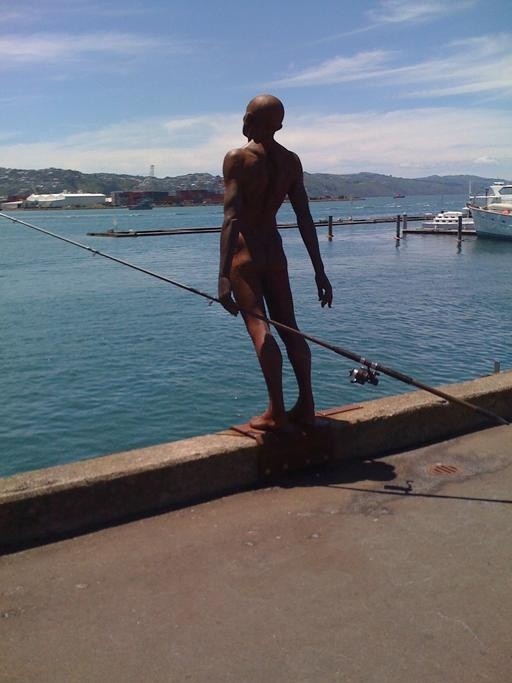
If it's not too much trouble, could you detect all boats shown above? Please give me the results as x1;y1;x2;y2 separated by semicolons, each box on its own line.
423;181;512;241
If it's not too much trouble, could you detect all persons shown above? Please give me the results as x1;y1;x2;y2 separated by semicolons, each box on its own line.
216;92;332;432
